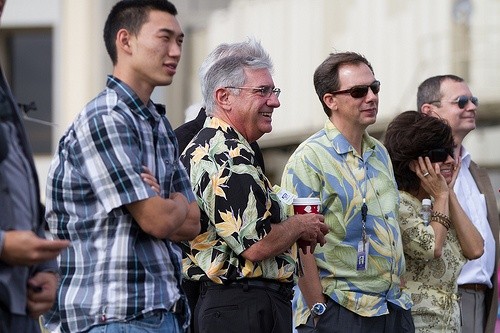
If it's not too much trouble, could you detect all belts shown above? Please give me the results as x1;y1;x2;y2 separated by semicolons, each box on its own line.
199;277;295;296
458;283;486;292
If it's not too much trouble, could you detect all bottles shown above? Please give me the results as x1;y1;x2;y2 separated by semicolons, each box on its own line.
419;199;433;228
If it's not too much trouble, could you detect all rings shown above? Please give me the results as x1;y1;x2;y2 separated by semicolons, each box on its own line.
423;172;429;177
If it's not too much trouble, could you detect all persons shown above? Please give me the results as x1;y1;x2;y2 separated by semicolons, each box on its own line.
384;111;485;332
45;0;202;332
172;101;266;178
177;41;329;333
280;51;416;333
0;0;69;333
416;74;499;333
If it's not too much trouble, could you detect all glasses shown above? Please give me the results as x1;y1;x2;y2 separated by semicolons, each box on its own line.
429;95;478;109
330;81;380;98
428;143;454;163
224;86;280;98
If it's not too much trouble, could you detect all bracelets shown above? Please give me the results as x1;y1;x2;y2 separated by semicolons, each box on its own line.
431;211;453;231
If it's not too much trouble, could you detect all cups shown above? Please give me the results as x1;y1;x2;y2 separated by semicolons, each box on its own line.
292;197;322;246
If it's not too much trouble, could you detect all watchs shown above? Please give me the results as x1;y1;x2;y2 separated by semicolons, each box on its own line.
308;302;332;316
